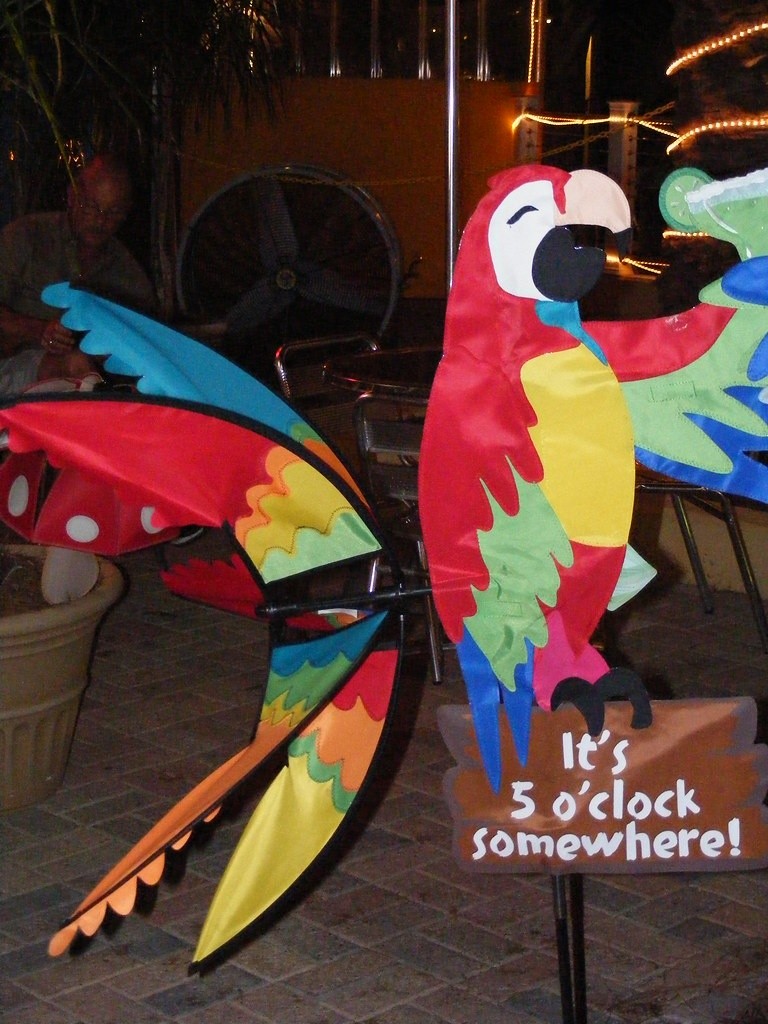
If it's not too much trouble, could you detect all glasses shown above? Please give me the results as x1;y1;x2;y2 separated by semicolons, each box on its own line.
75;195;126;219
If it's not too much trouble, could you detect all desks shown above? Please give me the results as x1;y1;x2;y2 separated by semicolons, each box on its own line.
321;343;449;402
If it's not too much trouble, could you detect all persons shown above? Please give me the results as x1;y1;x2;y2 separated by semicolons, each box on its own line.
0;145;157;397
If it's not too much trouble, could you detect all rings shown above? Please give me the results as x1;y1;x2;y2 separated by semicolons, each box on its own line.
49;340;54;345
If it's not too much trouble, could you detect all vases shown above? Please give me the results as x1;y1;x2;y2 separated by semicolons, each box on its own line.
0;542;123;812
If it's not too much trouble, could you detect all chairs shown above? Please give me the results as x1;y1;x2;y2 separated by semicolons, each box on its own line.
273;331;768;686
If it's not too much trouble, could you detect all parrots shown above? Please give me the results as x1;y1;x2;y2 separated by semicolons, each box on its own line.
415;160;768;794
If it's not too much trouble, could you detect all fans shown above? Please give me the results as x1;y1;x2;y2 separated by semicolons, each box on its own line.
174;164;403;371
